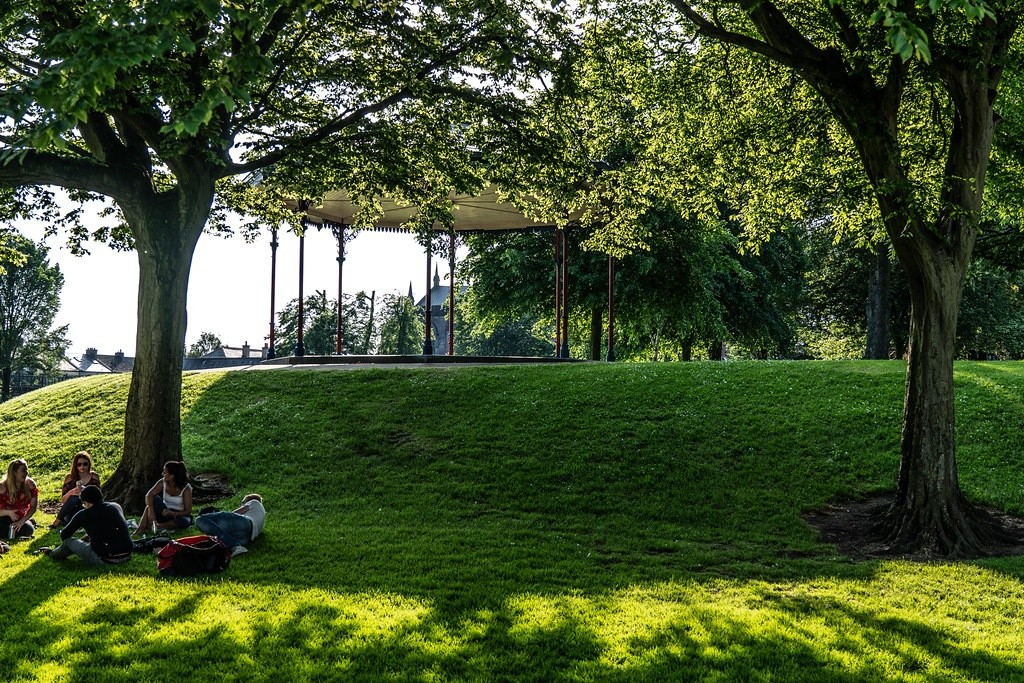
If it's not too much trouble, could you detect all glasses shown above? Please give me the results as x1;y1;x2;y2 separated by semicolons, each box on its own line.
15;460;22;463
77;462;88;466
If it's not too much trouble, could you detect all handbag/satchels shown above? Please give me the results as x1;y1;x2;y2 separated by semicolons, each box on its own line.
157;535;231;578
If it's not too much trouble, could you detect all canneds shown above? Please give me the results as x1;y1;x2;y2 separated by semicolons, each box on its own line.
8;524;15;539
76;480;83;493
152;520;158;534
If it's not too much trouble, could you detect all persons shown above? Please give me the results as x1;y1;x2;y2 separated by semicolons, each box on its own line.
0;450;265;568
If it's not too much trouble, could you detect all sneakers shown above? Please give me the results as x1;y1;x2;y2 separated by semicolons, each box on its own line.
231;546;248;558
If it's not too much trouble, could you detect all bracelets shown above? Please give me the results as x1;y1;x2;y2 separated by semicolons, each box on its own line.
147;504;153;508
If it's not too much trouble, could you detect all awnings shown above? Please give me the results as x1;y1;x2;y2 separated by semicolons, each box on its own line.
242;122;617;358
